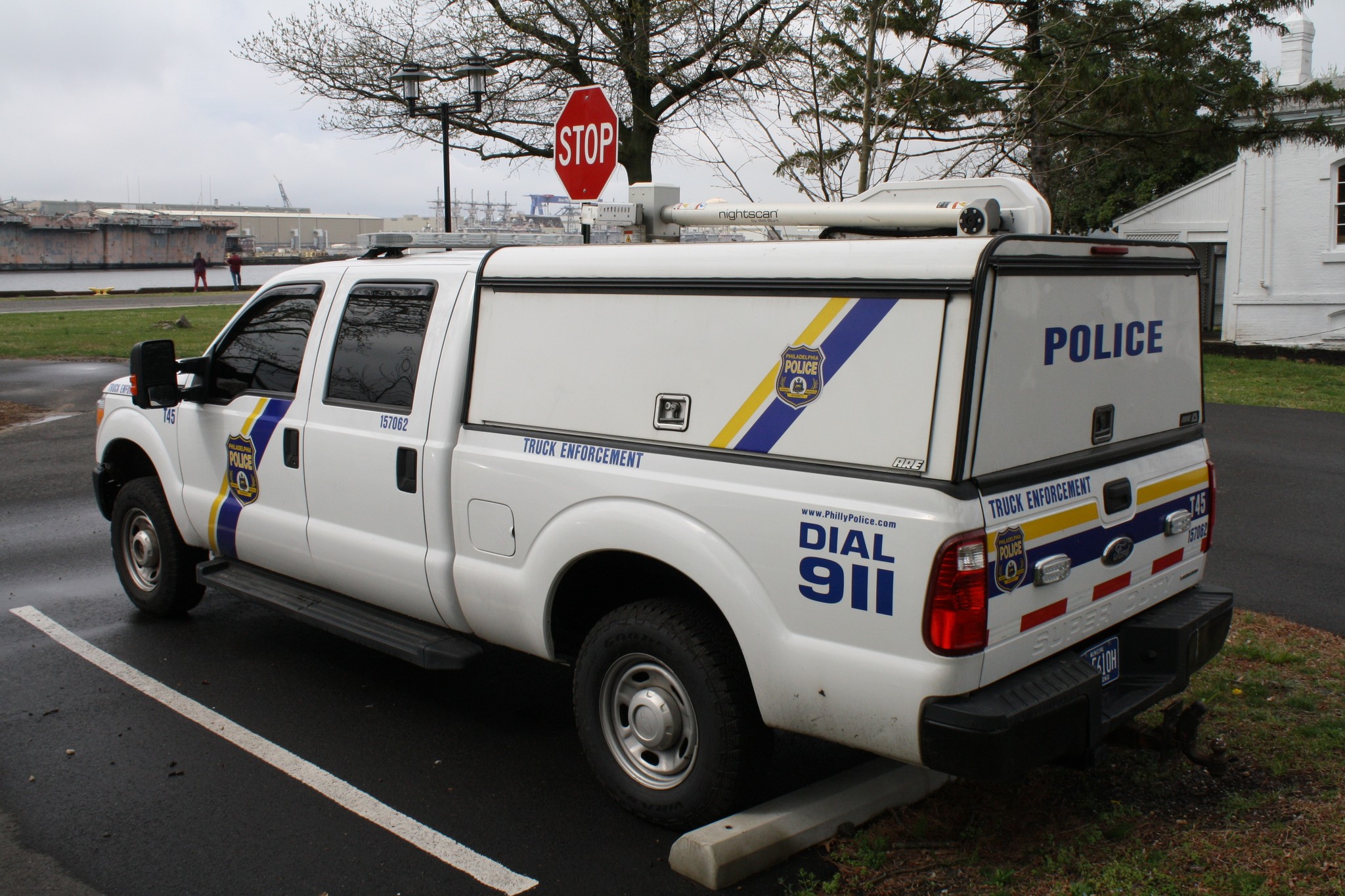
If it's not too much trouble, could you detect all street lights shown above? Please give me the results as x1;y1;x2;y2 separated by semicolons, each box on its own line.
390;50;499;253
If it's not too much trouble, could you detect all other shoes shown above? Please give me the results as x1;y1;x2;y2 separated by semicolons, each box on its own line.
206;290;208;292
193;290;197;292
232;286;241;291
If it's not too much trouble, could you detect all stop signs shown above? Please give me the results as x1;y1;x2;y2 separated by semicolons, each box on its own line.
553;85;619;202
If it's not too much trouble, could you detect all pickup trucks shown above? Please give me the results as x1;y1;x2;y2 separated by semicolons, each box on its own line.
90;177;1237;835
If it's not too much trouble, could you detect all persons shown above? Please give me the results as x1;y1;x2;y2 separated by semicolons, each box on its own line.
226;251;243;291
192;252;208;293
